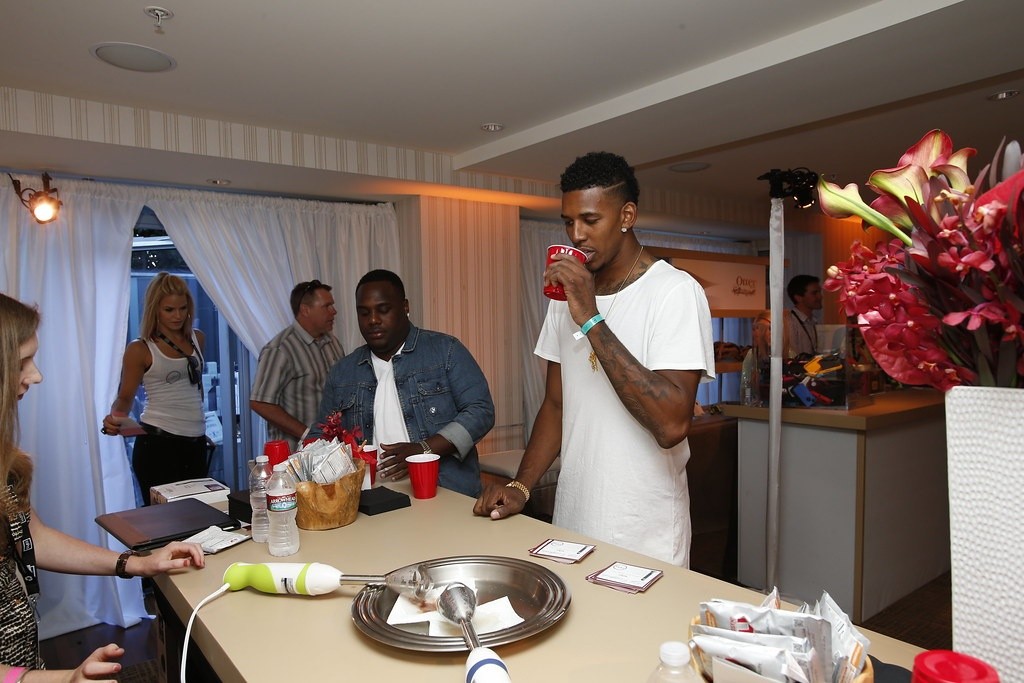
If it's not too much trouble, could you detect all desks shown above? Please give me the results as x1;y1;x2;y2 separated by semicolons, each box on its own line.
138;466;934;683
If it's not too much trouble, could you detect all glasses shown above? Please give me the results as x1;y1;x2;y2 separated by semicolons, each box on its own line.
298;280;320;305
187;356;202;384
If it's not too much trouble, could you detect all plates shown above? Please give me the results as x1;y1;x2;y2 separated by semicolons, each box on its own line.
351;555;572;652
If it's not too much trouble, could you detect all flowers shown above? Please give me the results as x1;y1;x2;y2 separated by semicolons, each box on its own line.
819;128;1024;393
315;410;364;444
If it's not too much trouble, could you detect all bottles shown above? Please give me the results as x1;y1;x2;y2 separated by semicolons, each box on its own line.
264;441;291;473
249;456;273;542
266;465;300;557
648;641;708;683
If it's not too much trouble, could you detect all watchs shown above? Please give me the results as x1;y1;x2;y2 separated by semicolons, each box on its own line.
419;440;432;454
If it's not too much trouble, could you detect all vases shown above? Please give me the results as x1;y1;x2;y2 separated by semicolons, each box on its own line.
944;385;1024;683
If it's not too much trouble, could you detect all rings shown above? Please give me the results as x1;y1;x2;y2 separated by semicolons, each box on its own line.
101;428;107;434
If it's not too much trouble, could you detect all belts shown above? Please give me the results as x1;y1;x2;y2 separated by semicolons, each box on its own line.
141;422;203;441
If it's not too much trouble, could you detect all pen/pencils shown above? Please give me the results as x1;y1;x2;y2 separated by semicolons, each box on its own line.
358;439;367;453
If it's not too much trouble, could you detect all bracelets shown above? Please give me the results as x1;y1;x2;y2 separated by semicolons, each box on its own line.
297;426;310;446
505;480;530;503
573;313;606;341
4;666;28;683
115;548;142;580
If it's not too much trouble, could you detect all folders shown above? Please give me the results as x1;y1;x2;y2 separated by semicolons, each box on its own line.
94;498;242;552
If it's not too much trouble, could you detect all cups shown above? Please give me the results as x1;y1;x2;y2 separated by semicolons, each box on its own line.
359;445;378;486
405;454;440;499
544;245;588;301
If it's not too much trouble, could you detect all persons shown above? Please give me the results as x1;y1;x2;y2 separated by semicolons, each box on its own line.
248;281;347;454
740;274;824;404
101;273;207;504
1;292;206;683
307;269;496;500
472;151;716;575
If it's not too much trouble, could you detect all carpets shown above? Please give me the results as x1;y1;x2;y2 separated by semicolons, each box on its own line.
98;658;160;683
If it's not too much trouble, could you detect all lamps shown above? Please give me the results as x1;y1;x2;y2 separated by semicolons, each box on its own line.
756;167;819;210
7;171;64;224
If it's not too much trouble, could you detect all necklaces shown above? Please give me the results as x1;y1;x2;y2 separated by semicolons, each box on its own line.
587;245;645;371
154;328;203;386
790;309;818;354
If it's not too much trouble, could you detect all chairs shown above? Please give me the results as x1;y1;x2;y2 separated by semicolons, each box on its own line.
477;449;561;516
